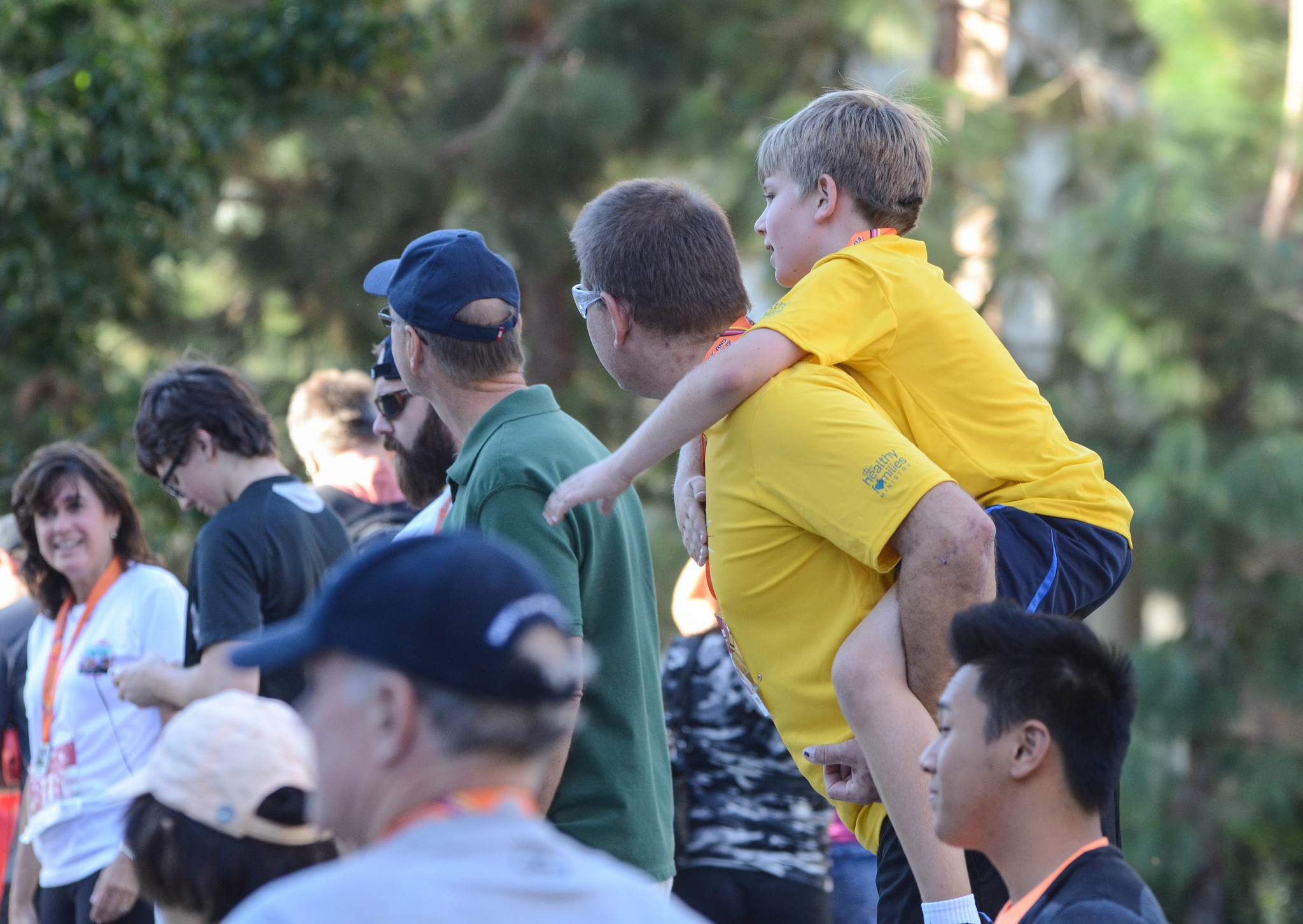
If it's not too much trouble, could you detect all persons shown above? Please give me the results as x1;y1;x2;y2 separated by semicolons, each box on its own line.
567;176;1011;924
919;599;1174;924
0;226;881;924
539;89;1136;924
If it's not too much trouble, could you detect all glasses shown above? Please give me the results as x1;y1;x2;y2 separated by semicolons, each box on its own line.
376;306;428;345
374;387;410;421
571;282;612;319
158;435;191;497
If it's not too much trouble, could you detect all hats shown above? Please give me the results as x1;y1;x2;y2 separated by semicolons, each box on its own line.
370;333;402;381
98;690;334;844
362;229;521;340
229;532;586;703
